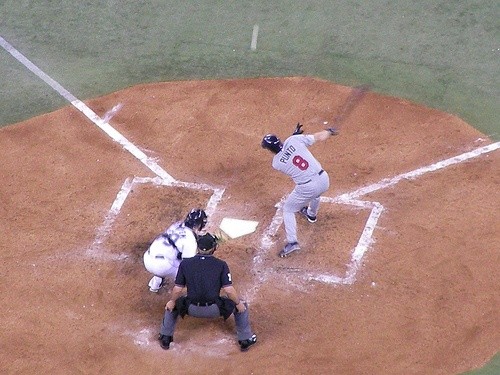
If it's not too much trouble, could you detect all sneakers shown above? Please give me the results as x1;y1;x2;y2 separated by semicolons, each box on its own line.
279;242;300;258
238;334;257;351
158;333;174;350
298;206;317;223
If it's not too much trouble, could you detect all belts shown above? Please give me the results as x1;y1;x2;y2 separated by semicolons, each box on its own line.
298;169;324;185
191;301;215;306
147;247;165;259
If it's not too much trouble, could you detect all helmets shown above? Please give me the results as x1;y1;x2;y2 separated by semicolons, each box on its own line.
184;208;207;230
197;232;219;255
262;133;281;148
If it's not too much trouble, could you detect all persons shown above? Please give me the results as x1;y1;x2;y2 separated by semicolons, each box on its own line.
141;206;210;293
258;120;341;258
155;228;258;353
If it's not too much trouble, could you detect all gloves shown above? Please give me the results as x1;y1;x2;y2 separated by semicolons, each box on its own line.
292;122;304;135
